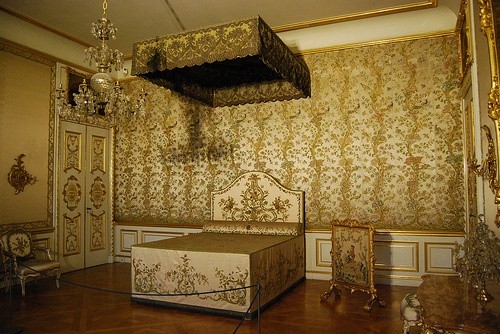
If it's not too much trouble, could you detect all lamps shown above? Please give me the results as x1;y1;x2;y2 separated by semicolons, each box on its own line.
452;214;500;303
52;0;147;129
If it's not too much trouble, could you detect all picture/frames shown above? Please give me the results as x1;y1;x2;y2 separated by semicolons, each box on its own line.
454;0;475;89
330;219;378;293
463;98;484;233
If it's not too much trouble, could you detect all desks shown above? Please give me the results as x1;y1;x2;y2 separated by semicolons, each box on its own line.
416;274;500;334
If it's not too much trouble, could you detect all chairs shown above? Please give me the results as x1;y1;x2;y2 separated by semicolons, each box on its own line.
1;227;61;295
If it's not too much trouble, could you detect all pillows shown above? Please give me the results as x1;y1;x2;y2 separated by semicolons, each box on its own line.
201;220;302;237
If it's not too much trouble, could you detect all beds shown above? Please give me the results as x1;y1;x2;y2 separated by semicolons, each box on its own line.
128;229;305;321
